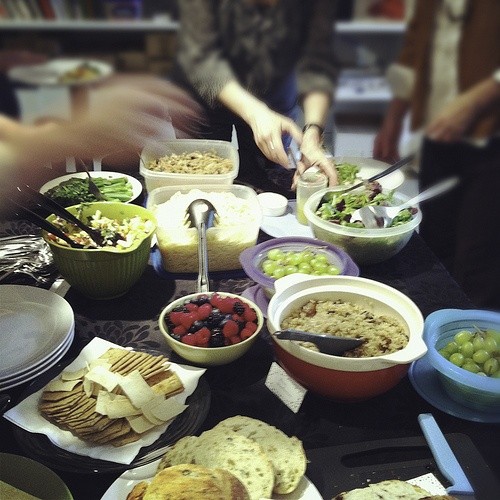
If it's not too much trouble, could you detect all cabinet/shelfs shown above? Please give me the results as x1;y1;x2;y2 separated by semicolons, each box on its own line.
0;0;413;160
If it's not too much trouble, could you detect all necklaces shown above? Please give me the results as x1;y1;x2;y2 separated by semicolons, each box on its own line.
447;3;464;24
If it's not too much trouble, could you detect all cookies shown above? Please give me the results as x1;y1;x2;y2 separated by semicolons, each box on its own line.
39;345;190;447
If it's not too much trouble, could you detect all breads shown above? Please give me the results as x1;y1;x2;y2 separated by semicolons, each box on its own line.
126;418;308;500
329;479;461;500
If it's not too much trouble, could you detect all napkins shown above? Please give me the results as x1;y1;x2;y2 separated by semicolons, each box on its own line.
3;334;206;467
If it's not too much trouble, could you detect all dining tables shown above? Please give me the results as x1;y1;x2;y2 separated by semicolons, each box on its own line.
0;164;500;500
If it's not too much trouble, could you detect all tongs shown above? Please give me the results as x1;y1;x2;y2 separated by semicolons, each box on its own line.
0;180;107;250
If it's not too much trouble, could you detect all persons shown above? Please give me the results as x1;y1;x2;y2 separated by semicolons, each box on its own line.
0;71;202;220
371;0;500;311
175;0;343;191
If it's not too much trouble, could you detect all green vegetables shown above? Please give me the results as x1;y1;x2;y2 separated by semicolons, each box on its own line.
42;177;133;207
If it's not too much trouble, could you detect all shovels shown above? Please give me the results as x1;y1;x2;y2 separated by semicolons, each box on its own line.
273;329;365;356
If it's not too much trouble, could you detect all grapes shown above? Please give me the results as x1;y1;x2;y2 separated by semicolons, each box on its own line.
439;322;499;381
261;249;340;279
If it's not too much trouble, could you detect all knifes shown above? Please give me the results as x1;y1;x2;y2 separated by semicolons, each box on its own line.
417;413;477;500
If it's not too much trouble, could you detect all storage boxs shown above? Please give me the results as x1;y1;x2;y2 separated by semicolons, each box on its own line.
136;136;240;194
146;183;263;274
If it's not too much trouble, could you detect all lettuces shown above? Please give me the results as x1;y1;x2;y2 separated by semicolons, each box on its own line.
314;163;414;263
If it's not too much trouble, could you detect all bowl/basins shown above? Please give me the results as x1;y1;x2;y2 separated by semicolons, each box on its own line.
304;185;423;266
146;184;261;272
239;236;360;300
139;138;240;195
426;308;500;412
266;272;428;399
257;192;288;217
159;292;264;367
41;202;157;299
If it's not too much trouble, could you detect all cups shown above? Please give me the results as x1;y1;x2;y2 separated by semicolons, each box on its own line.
296;172;327;226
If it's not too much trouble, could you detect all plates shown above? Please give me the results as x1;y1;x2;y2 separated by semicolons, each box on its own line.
409;356;500;424
260;199;313;239
100;458;323;500
39;169;142;203
0;285;212;500
6;56;113;86
305;155;405;190
241;283;269;318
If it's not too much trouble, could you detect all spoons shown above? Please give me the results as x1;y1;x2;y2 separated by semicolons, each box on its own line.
348;176;458;228
74;151;100;200
312;154;414;213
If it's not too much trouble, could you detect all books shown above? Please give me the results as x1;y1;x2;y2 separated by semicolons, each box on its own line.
0;0;101;21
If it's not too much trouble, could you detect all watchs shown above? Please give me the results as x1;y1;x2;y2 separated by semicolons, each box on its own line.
303;123;326;146
